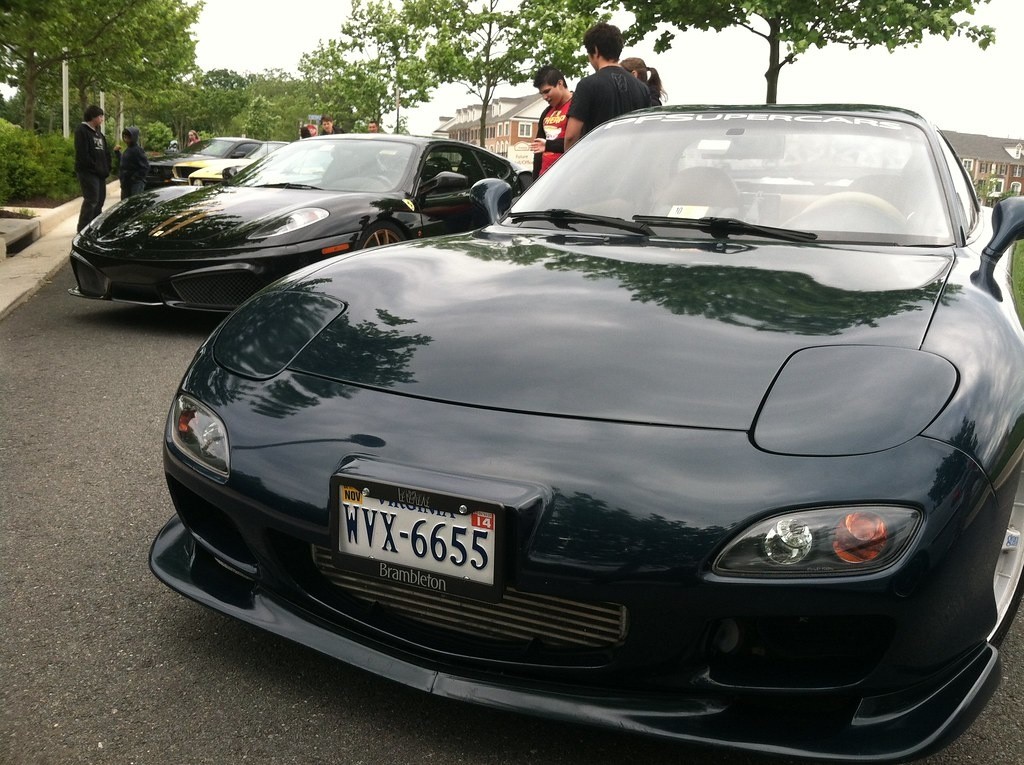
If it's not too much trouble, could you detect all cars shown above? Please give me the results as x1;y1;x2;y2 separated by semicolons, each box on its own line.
147;138;292;188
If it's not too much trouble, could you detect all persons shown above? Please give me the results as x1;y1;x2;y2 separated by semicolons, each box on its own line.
74;105;112;233
320;116;344;135
529;23;661;183
114;127;149;201
367;121;378;133
301;127;311;139
188;130;200;147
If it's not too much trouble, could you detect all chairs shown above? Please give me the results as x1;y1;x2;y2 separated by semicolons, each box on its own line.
844;173;906;211
419;157;453;183
653;165;741;215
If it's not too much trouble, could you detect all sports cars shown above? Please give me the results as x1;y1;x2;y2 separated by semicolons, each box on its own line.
148;101;1024;765
68;134;531;310
